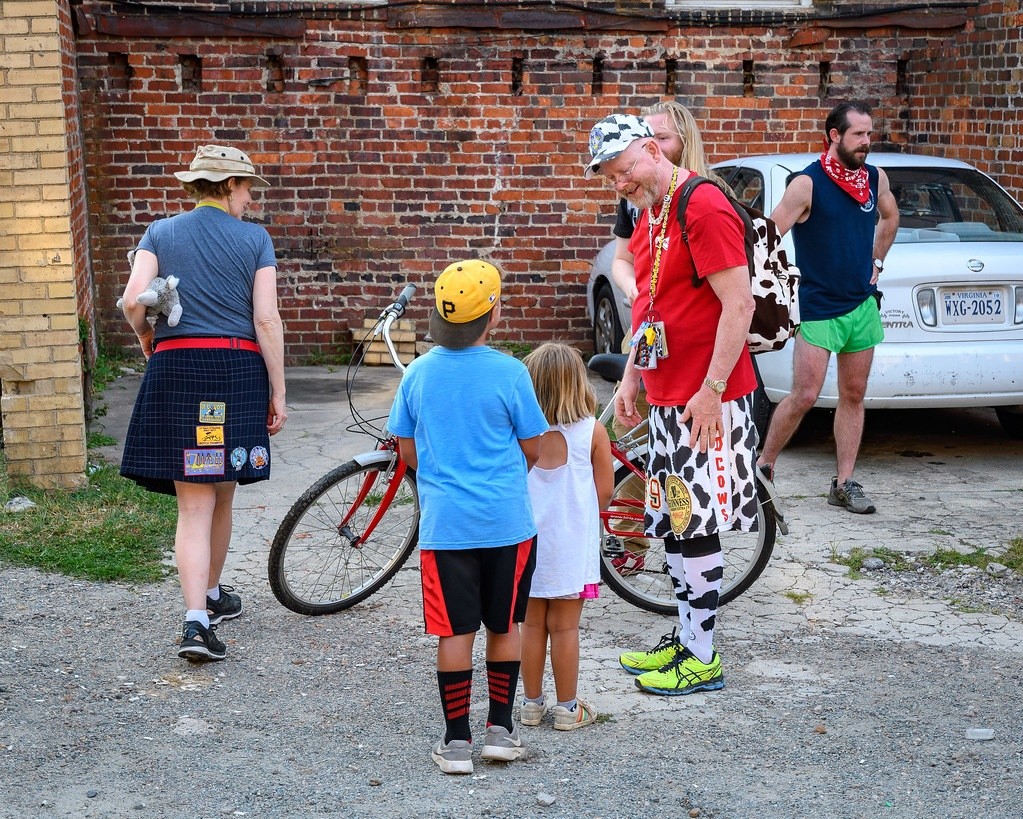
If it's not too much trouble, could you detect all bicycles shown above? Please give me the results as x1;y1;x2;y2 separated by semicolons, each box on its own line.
269;283;790;617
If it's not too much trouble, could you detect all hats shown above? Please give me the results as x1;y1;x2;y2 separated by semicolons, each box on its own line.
583;113;654;180
174;145;270;188
429;258;500;349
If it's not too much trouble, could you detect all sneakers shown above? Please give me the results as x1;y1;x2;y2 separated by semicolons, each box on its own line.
480;721;526;760
619;626;714;673
431;734;474;773
635;639;725;695
176;621;226;660
205;584;242;624
599;549;644;583
553;698;597;730
520;692;547;726
827;475;876;513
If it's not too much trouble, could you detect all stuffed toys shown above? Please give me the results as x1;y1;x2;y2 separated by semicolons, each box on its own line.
116;250;183;328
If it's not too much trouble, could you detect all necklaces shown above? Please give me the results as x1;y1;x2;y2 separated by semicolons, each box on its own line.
648;196;669;226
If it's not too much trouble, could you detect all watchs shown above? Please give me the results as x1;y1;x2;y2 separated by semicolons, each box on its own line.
872;258;883;273
703;377;728;394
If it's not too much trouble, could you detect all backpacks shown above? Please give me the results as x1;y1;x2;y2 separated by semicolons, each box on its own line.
677;175;802;354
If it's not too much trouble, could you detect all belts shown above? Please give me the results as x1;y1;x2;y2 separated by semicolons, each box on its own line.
152;337;262;352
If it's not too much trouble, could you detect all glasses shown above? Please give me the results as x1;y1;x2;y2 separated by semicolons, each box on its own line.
605;142;647;189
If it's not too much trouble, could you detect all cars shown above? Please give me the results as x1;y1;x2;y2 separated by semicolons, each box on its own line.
584;151;1023;449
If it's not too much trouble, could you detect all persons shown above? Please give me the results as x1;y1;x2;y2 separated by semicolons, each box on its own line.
584;102;759;695
755;99;900;515
520;341;615;731
385;258;549;774
119;145;288;662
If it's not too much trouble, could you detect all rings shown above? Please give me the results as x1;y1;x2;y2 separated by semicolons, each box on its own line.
709;428;715;431
281;426;284;429
